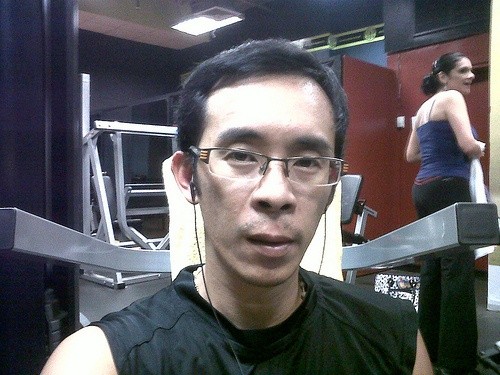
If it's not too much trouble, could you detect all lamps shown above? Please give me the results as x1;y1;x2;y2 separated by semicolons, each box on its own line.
168;0;247;37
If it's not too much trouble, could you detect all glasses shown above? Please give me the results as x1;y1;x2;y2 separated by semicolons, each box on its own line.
185;142;350;188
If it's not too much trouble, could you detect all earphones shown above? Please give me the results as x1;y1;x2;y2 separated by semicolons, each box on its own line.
189;174;196;202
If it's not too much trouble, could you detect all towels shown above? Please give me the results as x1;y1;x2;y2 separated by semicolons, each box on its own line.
468;140;495;259
162;146;345;284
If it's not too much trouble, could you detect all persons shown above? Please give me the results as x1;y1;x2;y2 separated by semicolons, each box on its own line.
405;52;486;374
36;38;434;375
